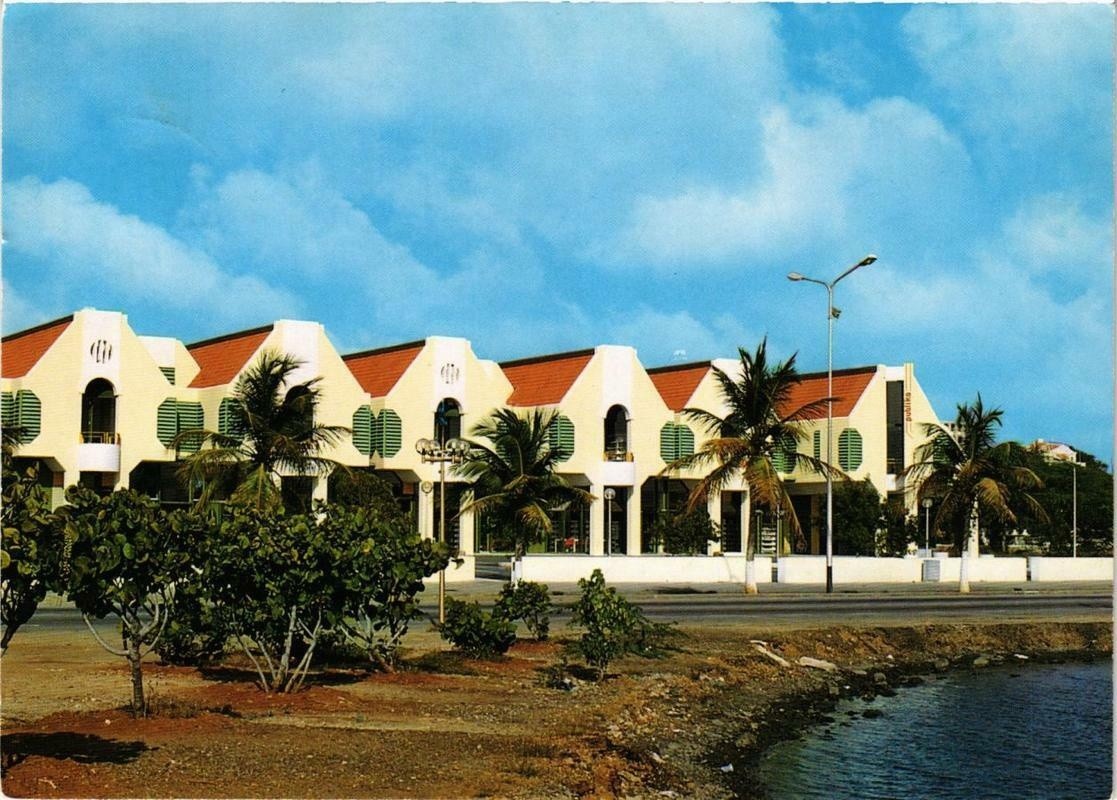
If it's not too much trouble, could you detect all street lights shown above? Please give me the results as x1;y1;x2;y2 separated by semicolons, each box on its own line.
416;438;471;625
921;498;932;559
784;253;879;596
604;488;615;558
